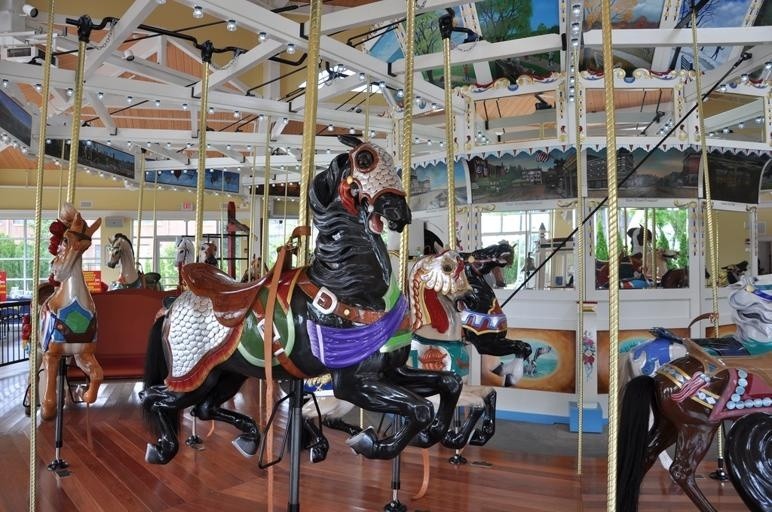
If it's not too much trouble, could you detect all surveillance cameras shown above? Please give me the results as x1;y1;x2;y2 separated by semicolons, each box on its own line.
17;4;39;17
